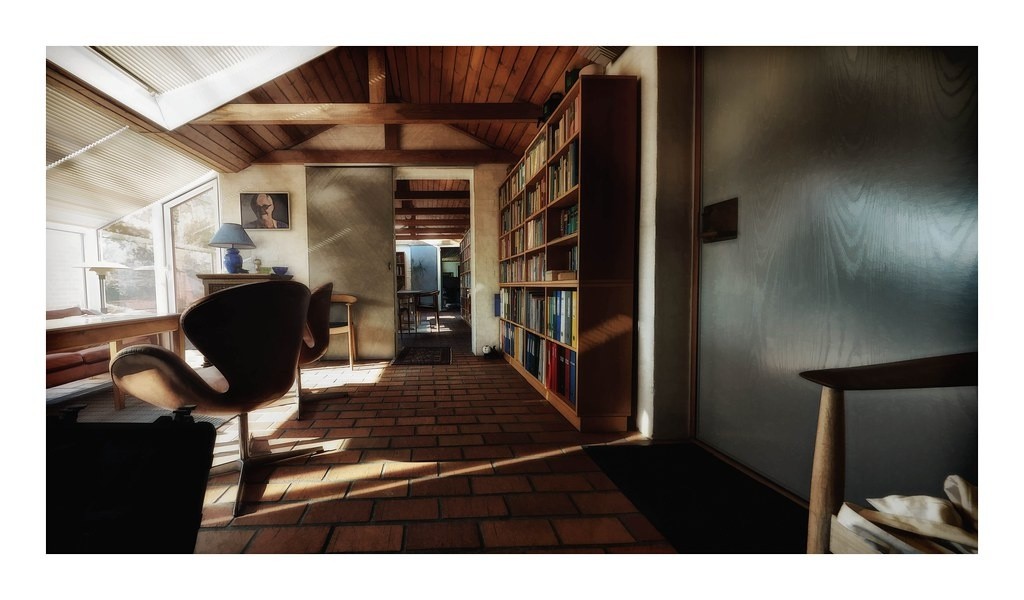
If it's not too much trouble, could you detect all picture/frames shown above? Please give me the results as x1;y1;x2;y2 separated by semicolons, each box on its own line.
240;192;290;230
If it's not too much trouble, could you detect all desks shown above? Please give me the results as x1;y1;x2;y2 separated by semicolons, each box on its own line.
46;313;185;410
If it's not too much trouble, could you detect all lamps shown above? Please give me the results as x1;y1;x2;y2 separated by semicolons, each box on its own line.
208;223;256;273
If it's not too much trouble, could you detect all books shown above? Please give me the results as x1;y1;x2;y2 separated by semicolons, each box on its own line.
497;92;581;410
396;252;406;291
459;228;472;325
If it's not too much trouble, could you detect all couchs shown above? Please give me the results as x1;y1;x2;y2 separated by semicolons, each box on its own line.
54;405;217;554
46;307;163;389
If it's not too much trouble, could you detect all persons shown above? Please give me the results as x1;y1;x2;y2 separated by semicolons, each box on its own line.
243;194;288;230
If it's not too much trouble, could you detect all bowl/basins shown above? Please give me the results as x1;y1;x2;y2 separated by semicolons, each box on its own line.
272;266;289;275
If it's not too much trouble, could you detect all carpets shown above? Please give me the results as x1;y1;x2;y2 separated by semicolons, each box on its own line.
392;346;453;365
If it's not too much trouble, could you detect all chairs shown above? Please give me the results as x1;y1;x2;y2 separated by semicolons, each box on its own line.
397;290;440;339
46;280;358;554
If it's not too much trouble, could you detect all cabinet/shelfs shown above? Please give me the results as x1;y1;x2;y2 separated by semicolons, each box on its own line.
499;74;641;432
195;274;294;297
460;228;472;328
396;252;408;328
